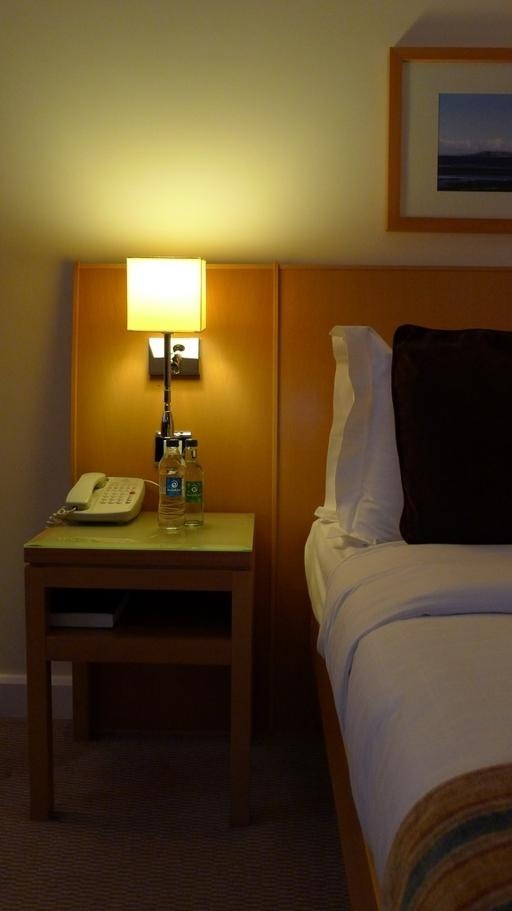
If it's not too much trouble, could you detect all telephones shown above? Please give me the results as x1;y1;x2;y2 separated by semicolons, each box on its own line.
66;472;147;526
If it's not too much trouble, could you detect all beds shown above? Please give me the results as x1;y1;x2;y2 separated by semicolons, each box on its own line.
301;518;512;911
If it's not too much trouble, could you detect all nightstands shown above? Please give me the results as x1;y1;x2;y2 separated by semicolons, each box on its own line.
21;513;257;821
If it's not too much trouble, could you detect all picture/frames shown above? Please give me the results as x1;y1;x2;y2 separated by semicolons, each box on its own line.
384;44;512;235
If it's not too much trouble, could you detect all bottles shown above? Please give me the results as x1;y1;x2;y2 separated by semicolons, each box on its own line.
183;438;206;528
157;438;184;532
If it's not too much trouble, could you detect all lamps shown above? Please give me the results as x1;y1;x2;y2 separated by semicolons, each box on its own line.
123;253;210;469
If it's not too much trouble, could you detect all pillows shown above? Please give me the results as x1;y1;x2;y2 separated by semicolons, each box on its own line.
314;327;511;547
